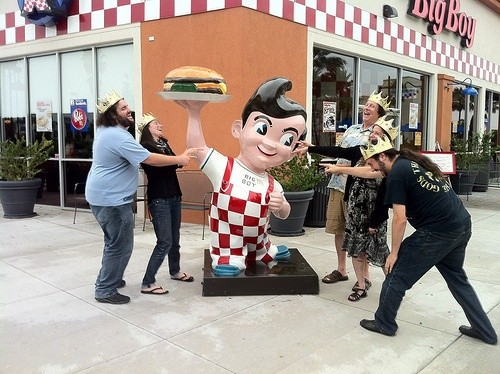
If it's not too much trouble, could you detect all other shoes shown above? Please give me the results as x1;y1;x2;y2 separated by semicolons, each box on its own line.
360;319;394;336
459;324;498;344
95;292;131;304
116;280;125;288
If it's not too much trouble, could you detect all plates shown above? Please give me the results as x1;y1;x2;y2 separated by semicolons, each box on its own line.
158;91;232;104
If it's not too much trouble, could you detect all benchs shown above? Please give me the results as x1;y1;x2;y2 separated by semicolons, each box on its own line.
142;189;212;241
73;181;147;230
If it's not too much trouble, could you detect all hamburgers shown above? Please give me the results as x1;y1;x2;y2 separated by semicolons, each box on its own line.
162;66;227;95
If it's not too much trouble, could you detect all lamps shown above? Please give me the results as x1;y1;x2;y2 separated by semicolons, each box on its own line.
383;3;399;19
444;77;479;97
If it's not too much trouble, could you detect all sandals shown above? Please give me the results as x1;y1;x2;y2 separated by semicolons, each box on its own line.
348;287;368;302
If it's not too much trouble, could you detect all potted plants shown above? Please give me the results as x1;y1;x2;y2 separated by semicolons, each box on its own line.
448;130;500;195
266;154;326;238
0;135;56;220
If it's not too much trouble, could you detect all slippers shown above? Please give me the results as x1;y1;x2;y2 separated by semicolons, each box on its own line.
141;285;169;295
171;272;194;282
352;277;372;292
322;270;349;284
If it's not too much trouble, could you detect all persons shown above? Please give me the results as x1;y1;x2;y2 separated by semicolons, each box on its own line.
135;113;203;294
174;78;307;275
86;90;190;304
289;92;499;344
